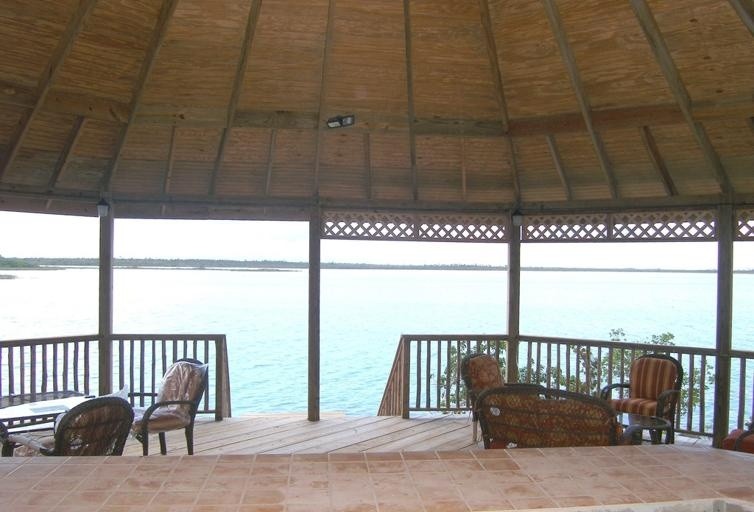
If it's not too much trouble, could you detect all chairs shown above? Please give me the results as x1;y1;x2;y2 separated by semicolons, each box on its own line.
461;353;544;444
7;357;208;455
599;354;683;444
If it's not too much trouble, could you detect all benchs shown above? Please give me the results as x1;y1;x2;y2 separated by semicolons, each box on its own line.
475;386;642;449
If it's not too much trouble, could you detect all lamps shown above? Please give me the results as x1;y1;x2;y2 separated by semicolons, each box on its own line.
326;115;357;130
